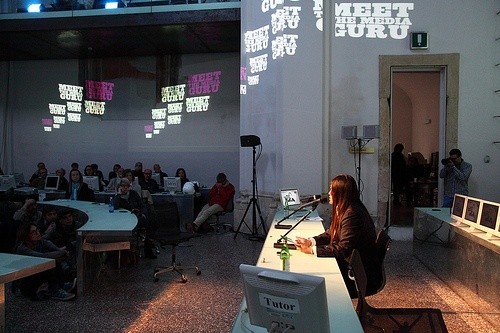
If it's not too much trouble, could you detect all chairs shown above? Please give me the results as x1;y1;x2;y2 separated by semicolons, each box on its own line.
208;202;233;235
351;227;391;322
146;201;201;284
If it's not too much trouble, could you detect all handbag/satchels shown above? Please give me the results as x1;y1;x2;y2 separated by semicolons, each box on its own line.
144;238;166;259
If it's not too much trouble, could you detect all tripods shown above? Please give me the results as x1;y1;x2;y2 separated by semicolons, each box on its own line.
233;147;268;241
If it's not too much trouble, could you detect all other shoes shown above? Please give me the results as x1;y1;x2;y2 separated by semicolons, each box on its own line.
186;223;195;233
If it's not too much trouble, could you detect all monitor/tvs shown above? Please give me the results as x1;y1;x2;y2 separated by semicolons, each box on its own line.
151;173;161;185
164;177;181;195
83;176;99;191
239;263;330;333
44;176;60;191
0;172;26;190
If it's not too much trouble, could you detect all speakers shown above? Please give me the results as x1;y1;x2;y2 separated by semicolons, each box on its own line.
341;125;358;139
240;135;261;147
363;125;380;138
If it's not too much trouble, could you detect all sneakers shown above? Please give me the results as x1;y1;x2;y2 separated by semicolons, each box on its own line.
62;278;76;293
51;289;75;300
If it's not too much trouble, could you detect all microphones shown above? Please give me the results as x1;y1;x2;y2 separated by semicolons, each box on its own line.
300;194;329;207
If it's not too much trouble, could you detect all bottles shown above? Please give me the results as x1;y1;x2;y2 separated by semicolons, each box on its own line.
109;197;114;213
279;237;291;272
284;197;289;219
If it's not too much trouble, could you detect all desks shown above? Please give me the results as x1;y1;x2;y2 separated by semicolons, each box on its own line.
413;207;500;333
231;203;365;333
0;186;201;333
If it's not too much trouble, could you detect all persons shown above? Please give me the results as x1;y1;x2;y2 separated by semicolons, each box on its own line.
295;174;377;290
391;144;472;210
0;161;190;301
186;173;235;233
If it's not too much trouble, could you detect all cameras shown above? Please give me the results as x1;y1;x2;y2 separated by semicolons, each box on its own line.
441;157;455;165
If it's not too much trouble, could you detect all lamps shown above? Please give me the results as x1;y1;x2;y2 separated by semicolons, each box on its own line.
342;124;380;140
240;135;261;147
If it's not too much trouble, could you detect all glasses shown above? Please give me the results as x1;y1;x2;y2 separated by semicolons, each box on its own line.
29;227;40;235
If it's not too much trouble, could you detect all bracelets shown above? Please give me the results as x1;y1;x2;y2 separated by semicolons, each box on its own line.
451;165;455;169
